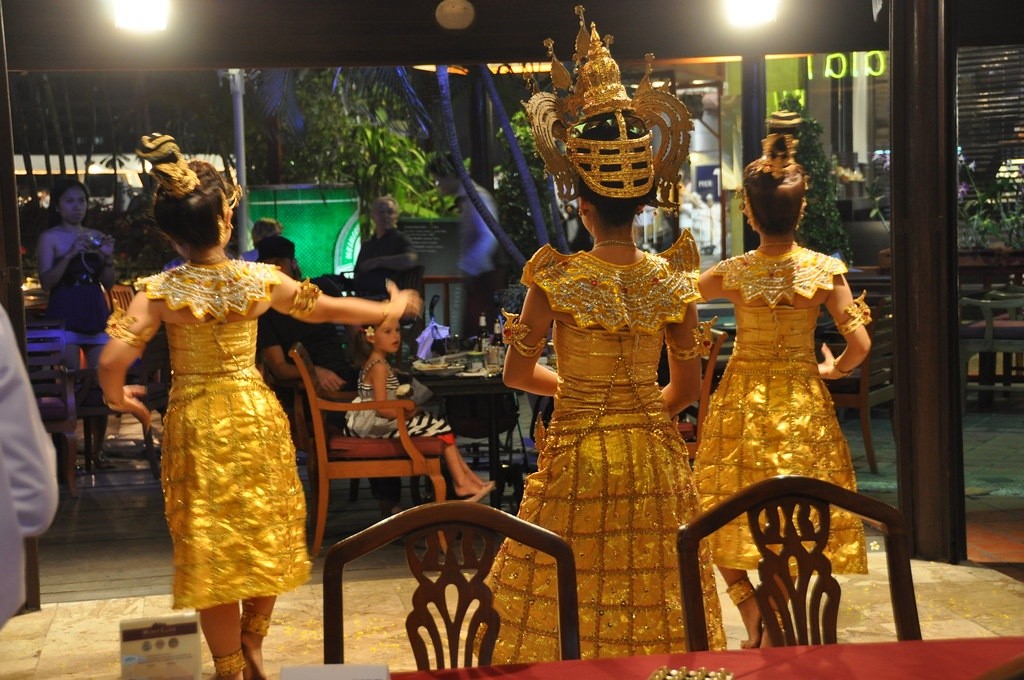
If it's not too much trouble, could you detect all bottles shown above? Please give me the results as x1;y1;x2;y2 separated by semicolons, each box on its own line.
466;351;485;373
477;311;490;367
491;319;506;367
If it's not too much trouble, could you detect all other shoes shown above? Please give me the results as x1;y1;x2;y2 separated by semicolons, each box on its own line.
71;465;87;476
464;480;496;504
93;452;114;469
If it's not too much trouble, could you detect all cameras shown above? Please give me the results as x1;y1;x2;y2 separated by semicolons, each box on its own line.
85;235;102;251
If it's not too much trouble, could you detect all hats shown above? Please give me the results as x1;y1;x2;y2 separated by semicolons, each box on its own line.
254;235;295;262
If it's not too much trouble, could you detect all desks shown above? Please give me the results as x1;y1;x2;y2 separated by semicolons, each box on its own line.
407;374;524;509
389;636;1024;680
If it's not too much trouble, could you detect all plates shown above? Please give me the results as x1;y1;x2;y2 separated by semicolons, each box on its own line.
414;364;465;376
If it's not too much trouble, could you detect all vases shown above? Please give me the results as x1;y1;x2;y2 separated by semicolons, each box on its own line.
876;244;1020;283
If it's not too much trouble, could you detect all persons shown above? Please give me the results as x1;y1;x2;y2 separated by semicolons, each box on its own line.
1;297;60;629
238;153;509;518
696;131;874;646
95;131;426;678
35;178;118;470
463;106;729;668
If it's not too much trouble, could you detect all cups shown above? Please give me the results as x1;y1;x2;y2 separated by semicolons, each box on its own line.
444;334;460;353
485;346;500;373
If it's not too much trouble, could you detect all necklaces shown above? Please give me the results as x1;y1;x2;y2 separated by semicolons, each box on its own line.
758;241;798;249
593;240;639;247
190;254;229;266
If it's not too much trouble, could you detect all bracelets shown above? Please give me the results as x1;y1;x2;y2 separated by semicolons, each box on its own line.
102;392;132;410
832;355;854;378
102;259;114;267
380;297;390;326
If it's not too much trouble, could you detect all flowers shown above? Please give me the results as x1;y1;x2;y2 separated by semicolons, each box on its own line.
864;143;1024;252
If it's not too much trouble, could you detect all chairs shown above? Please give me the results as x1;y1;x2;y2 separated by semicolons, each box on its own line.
676;475;923;651
26;267;1024;559
322;501;581;672
